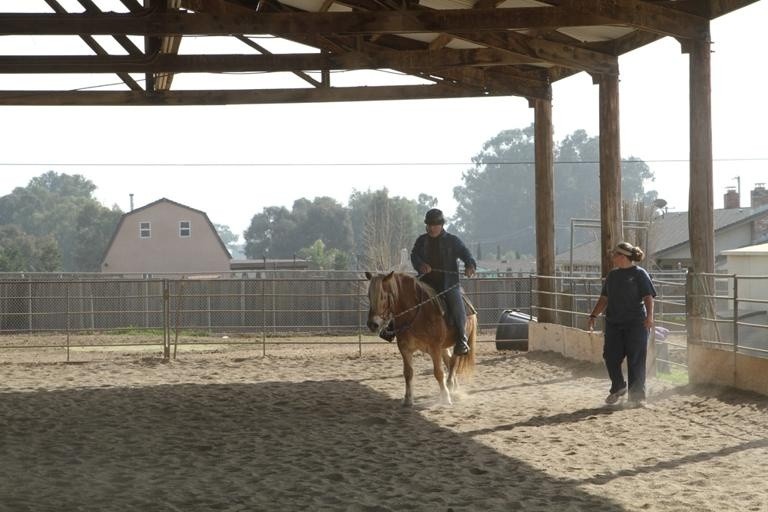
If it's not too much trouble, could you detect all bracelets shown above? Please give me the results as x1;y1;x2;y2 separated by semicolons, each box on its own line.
590;315;597;318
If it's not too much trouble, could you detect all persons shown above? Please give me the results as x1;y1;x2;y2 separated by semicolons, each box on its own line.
588;241;657;409
380;209;476;354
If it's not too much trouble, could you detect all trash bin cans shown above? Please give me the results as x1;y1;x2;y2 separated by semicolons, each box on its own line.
494;305;538;351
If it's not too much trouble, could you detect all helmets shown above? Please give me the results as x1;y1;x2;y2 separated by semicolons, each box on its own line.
423;209;444;225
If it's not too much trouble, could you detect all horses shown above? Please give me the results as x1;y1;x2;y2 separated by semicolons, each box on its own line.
365;269;478;409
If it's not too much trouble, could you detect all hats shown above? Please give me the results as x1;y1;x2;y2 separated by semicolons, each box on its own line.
605;245;633;257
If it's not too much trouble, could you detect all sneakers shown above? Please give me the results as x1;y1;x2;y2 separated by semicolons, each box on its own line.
379;327;395;341
604;387;626;404
453;338;467;355
622;400;645;410
